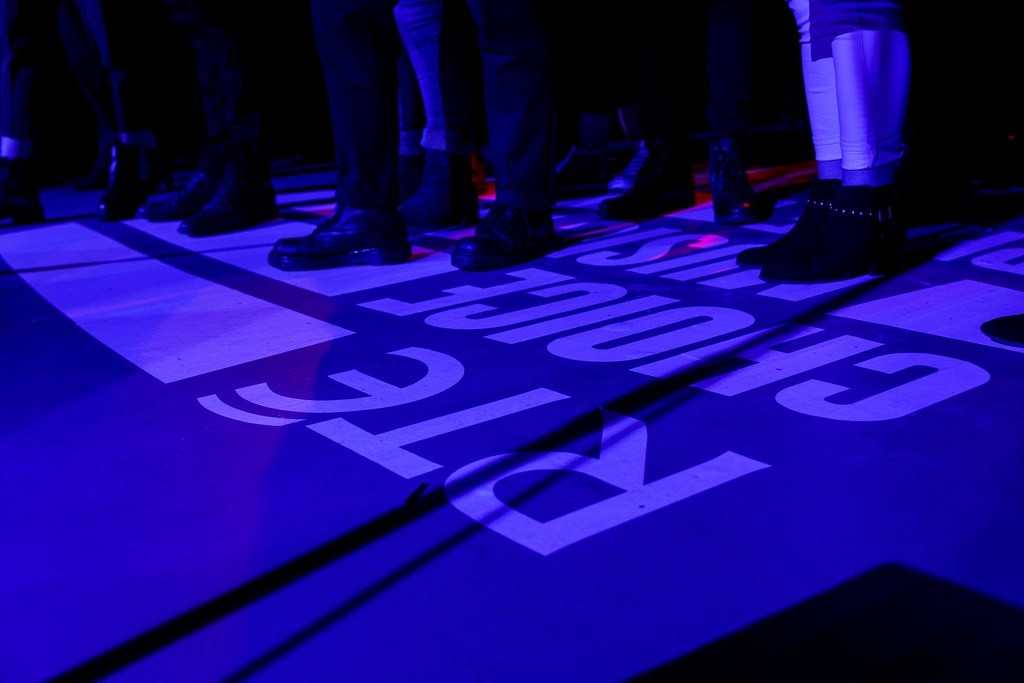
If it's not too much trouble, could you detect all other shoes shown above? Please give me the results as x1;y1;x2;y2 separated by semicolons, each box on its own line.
0;155;44;227
707;151;772;225
98;144;175;222
397;152;478;227
597;159;694;221
551;147;608;198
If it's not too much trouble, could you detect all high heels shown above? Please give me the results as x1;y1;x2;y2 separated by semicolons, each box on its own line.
734;177;915;288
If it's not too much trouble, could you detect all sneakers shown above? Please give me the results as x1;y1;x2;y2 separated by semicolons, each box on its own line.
141;173;278;236
450;206;564;271
267;207;414;273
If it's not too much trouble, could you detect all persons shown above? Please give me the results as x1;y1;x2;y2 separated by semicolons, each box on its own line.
1;0;913;285
